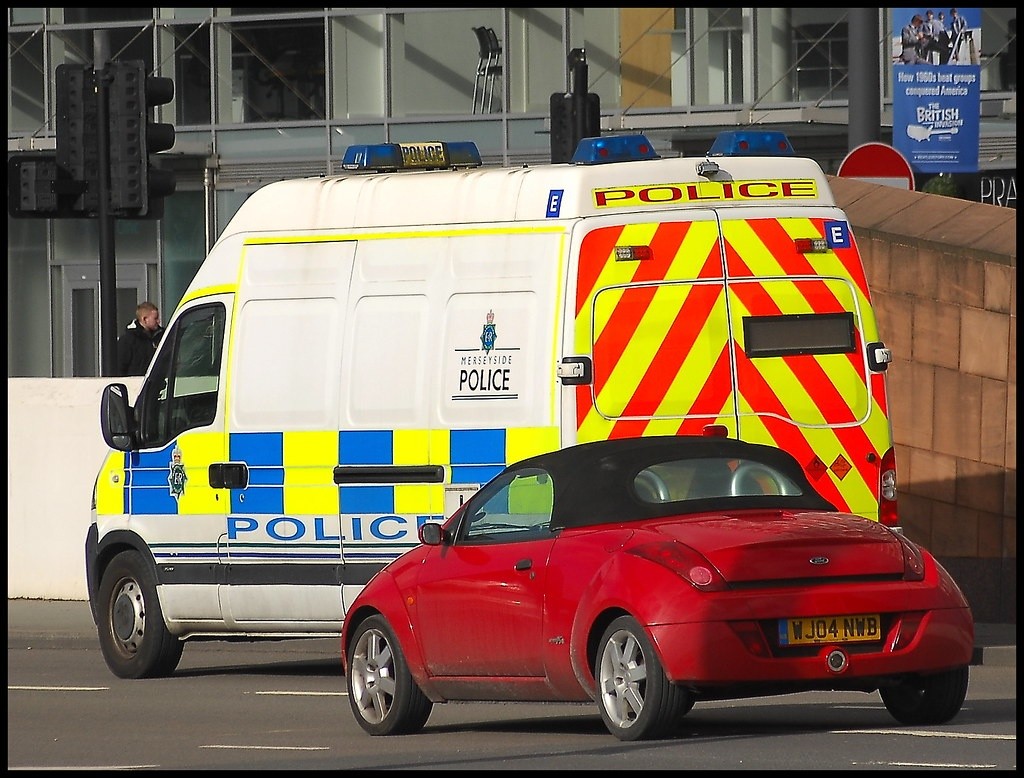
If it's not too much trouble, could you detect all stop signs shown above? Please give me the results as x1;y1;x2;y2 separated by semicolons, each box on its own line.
833;142;913;194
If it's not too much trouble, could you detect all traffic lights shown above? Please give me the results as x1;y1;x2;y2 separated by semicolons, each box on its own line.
54;62;175;220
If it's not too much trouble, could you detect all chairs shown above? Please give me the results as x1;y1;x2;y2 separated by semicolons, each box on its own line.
470;25;504;114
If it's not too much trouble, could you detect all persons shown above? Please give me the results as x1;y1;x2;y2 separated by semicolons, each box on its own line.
115;302;168;376
898;8;968;65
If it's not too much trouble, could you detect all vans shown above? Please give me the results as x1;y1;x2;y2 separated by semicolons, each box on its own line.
77;125;902;680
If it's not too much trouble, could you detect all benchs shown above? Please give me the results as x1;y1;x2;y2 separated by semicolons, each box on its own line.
632;459;787;504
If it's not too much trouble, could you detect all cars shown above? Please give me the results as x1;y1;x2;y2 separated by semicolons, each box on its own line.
339;438;980;743
